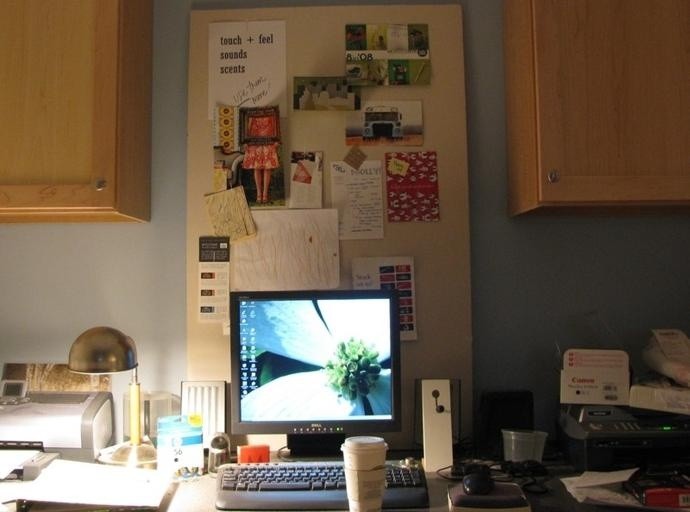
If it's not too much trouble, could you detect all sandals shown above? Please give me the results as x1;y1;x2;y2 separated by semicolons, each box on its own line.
256;195;270;203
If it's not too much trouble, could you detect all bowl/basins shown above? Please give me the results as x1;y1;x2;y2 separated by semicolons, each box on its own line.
501;427;549;464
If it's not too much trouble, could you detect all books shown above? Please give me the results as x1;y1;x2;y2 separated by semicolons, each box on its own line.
623;467;690;508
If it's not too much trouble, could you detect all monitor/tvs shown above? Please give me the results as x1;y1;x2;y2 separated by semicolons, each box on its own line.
229;290;401;458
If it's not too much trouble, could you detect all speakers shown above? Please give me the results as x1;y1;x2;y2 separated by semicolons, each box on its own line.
414;379;461;473
473;387;534;458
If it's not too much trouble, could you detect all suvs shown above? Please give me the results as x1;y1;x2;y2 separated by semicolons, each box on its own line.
361;105;405;141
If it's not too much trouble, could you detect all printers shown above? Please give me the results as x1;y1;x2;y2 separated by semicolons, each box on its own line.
0;380;114;479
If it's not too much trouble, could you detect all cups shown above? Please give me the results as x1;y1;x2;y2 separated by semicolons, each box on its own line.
340;435;390;512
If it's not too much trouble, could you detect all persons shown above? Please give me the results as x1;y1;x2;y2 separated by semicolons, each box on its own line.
242;112;280;202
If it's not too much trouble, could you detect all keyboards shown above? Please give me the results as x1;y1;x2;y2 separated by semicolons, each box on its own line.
215;462;429;510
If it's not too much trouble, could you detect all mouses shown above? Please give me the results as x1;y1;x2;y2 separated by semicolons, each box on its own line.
463;472;491;496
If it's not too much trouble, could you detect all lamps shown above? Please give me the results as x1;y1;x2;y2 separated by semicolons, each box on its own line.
68;325;159;468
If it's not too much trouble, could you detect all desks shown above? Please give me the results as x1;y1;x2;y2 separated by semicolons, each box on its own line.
5;442;688;512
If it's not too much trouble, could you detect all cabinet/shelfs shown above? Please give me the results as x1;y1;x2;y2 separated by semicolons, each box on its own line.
0;2;150;222
497;1;690;219
